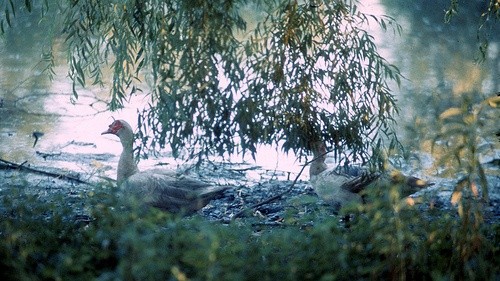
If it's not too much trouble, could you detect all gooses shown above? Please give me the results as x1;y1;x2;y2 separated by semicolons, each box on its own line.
304;138;435;229
101;119;233;216
